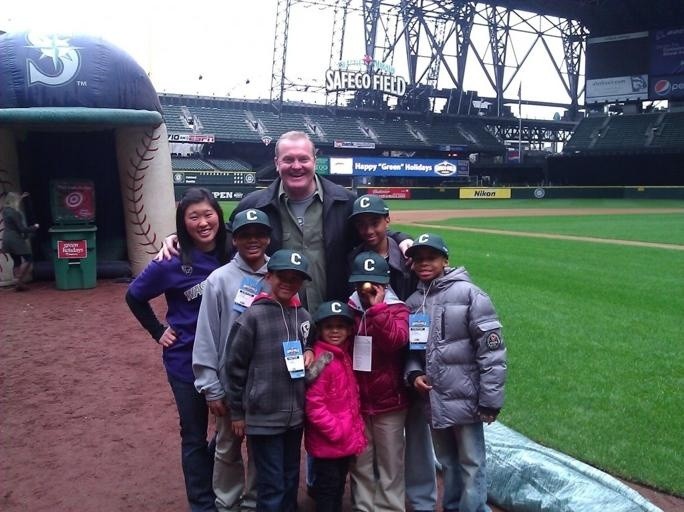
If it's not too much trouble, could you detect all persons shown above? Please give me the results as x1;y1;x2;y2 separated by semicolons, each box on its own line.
345;195;442;511
346;251;411;511
154;131;414;500
398;233;507;511
191;208;300;512
125;189;237;512
303;300;369;512
224;249;312;512
2;192;39;292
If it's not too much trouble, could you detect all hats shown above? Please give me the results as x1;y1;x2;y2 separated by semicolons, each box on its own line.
348;194;389;219
314;301;353;323
232;208;272;234
349;251;389;284
267;249;313;281
405;234;448;257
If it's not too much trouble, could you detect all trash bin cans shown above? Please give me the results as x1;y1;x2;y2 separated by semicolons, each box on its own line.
49;179;98;290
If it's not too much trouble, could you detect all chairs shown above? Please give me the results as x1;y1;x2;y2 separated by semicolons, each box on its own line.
562;112;683;155
155;96;508;152
